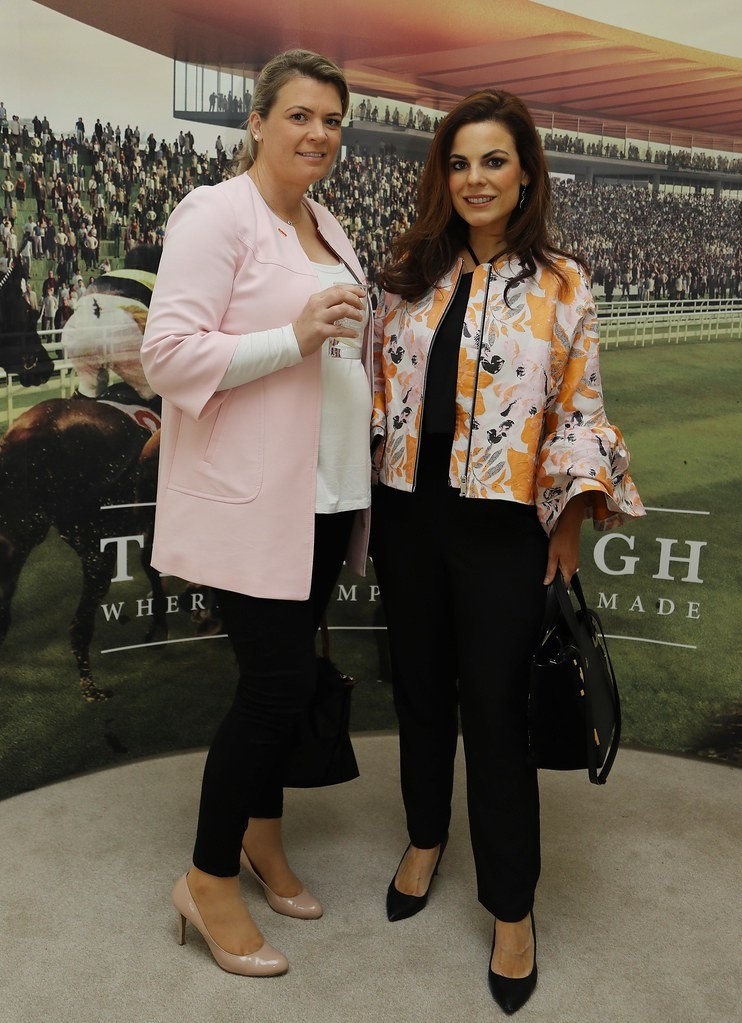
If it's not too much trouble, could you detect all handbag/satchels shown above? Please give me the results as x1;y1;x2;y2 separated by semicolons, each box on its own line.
529;566;624;786
281;614;359;788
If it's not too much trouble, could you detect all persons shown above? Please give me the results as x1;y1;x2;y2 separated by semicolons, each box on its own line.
372;88;648;1016
0;88;742;489
139;46;371;977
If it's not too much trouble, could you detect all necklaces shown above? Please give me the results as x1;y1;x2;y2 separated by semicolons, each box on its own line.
260;194;303;227
466;242;510;266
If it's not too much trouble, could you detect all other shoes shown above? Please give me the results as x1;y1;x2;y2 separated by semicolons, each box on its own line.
488;904;539;1016
386;831;450;922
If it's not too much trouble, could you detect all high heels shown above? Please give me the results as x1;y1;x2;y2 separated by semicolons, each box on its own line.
240;840;323;921
173;870;289;978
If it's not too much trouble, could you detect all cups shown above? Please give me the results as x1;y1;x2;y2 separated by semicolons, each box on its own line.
327;281;370;360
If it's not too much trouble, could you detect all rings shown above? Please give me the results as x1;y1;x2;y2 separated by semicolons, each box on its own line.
576;568;580;573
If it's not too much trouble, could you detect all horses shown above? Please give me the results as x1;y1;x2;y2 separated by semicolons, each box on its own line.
0;381;206;703
1;255;57;387
